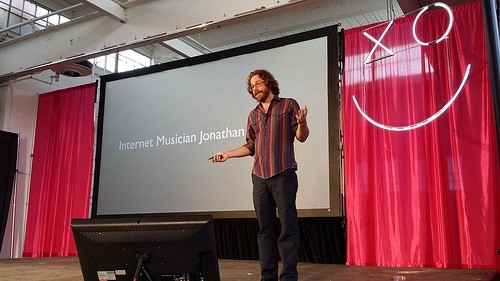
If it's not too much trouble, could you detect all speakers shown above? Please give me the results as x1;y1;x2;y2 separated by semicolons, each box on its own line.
1;130;19;249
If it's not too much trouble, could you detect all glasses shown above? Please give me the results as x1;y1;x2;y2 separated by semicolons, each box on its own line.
250;81;266;91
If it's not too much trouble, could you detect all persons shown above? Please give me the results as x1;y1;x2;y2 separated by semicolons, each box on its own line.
212;70;310;281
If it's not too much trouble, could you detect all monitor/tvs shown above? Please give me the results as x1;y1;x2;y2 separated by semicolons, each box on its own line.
71;214;220;281
